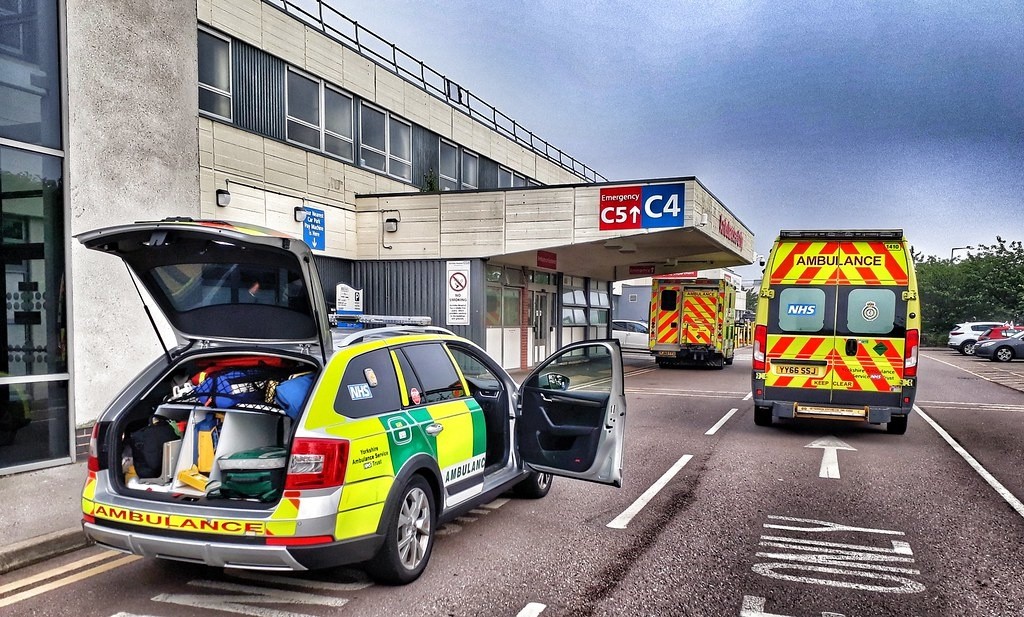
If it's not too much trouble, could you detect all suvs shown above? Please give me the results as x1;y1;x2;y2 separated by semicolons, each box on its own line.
978;325;1024;342
947;321;1021;356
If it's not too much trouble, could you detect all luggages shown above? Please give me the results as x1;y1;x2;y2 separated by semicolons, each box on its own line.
218;445;288;503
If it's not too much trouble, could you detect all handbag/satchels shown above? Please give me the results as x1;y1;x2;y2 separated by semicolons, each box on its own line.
127;414;179;477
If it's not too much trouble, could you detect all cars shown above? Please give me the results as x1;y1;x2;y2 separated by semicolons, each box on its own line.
973;330;1024;363
611;319;650;352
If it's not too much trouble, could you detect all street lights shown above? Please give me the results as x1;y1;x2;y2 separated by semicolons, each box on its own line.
951;247;974;263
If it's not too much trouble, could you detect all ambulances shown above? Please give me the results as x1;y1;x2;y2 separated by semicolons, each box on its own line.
70;215;627;587
648;278;745;371
750;229;921;435
